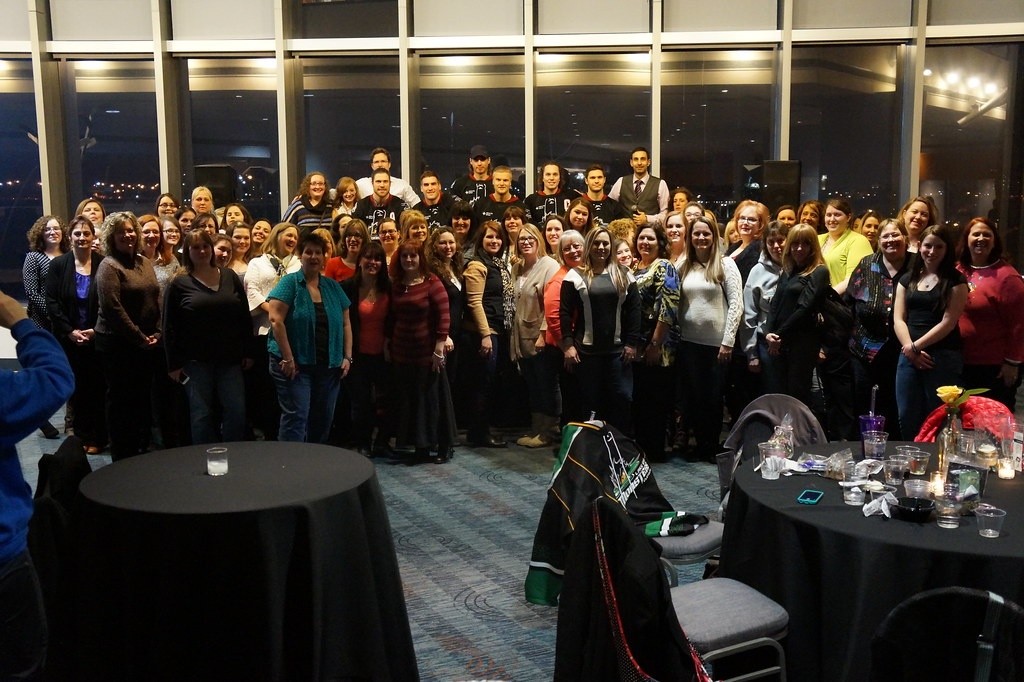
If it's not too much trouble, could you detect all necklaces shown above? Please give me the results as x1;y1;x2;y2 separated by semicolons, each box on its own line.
403;281;409;293
912;244;914;246
923;278;933;288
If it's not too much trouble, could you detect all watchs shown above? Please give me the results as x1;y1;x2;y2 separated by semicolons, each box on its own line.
648;340;660;347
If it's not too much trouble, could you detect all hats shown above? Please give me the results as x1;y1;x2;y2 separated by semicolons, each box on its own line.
493;155;510;167
471;145;487;159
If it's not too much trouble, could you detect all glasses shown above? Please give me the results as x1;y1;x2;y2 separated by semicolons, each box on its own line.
159;204;176;208
737;216;760;223
310;182;325;186
684;212;702;217
518;238;534;243
346;234;363;238
162;229;181;234
378;230;396;234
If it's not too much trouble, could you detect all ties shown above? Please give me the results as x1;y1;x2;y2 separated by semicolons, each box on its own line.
635;181;642;198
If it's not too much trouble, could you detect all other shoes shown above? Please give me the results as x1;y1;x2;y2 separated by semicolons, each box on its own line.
517;434;552;447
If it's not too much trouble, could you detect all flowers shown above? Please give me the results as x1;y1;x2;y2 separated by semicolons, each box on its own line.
936;383;989;416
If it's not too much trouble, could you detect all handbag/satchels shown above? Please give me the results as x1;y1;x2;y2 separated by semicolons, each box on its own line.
806;265;854;350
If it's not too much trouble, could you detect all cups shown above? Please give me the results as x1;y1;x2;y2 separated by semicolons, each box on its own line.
936;494;962;528
895;445;920;457
907;451;930;476
975;507;1006;537
944;483;960;497
206;447;229;476
956;434;974;462
882;461;905;486
998;454;1016;480
904;480;932;501
889;455;913;479
758;443;785;481
858;415;885;458
862;430;889;460
869;483;897;514
841;466;870;506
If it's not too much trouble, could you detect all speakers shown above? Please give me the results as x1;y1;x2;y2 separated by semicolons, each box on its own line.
760;159;802;221
193;165;238;209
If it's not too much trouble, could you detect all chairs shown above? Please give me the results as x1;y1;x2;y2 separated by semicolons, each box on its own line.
871;589;1024;682
913;397;1018;452
572;420;724;586
716;394;827;520
593;495;788;682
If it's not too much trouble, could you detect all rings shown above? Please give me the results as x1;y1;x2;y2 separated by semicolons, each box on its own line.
725;357;727;359
432;370;435;371
443;362;445;365
441;365;443;366
632;356;635;358
1014;376;1016;379
919;366;923;369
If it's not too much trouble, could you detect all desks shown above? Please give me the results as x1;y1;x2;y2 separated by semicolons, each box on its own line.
726;442;1024;682
79;442;419;682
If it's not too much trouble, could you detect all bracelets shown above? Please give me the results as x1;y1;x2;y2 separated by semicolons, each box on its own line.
282;356;294;363
911;342;918;355
433;351;445;359
912;342;920;353
344;354;353;364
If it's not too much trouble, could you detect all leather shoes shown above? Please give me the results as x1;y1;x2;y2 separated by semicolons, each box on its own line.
466;432;507;447
373;444;403;458
434;447;453;463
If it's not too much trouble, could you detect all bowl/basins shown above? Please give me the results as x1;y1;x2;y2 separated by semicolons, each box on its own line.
893;497;936;521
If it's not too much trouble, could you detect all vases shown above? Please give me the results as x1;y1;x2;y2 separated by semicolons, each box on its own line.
936;415;957;482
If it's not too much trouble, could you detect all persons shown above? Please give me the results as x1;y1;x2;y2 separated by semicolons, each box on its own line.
517;173;525;195
561;167;584;201
1;287;76;682
987;200;998;224
24;146;1024;466
486;155;527;201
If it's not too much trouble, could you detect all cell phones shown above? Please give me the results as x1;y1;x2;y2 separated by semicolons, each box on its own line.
179;372;190;385
798;490;824;504
